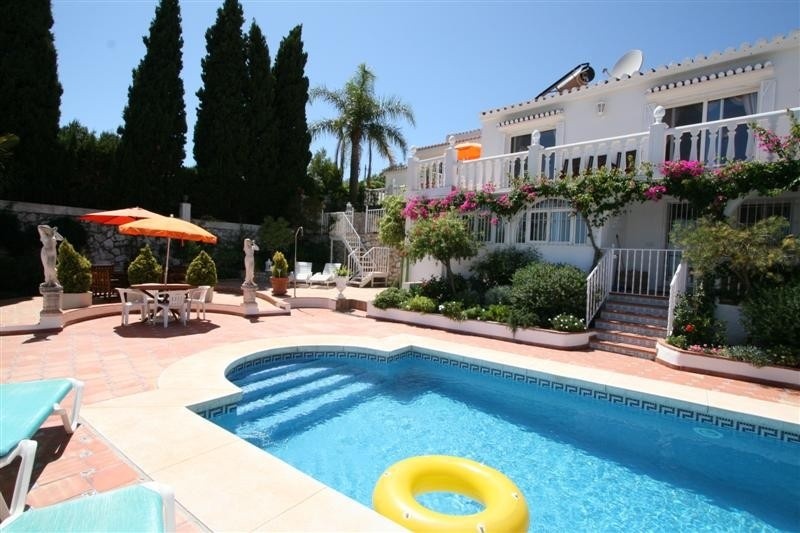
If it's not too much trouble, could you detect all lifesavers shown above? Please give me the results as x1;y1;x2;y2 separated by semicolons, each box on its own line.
371;455;529;533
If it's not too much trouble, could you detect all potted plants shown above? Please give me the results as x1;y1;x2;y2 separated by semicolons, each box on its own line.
269;251;290;294
126;243;163;305
333;265;353;300
55;238;94;311
185;250;218;302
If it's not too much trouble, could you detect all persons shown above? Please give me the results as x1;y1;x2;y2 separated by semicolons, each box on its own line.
243;238;259;287
38;225;64;287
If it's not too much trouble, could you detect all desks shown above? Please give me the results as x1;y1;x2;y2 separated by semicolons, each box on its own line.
131;283;192;323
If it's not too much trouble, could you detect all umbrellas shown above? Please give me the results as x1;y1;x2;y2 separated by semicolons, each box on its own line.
80;206;217;284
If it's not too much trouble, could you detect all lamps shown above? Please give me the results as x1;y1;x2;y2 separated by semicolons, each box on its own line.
596;100;608;116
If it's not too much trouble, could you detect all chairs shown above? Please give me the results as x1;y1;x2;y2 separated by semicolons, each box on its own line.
92;270;120;303
0;376;179;533
308;262;342;288
115;282;211;330
289;260;313;289
